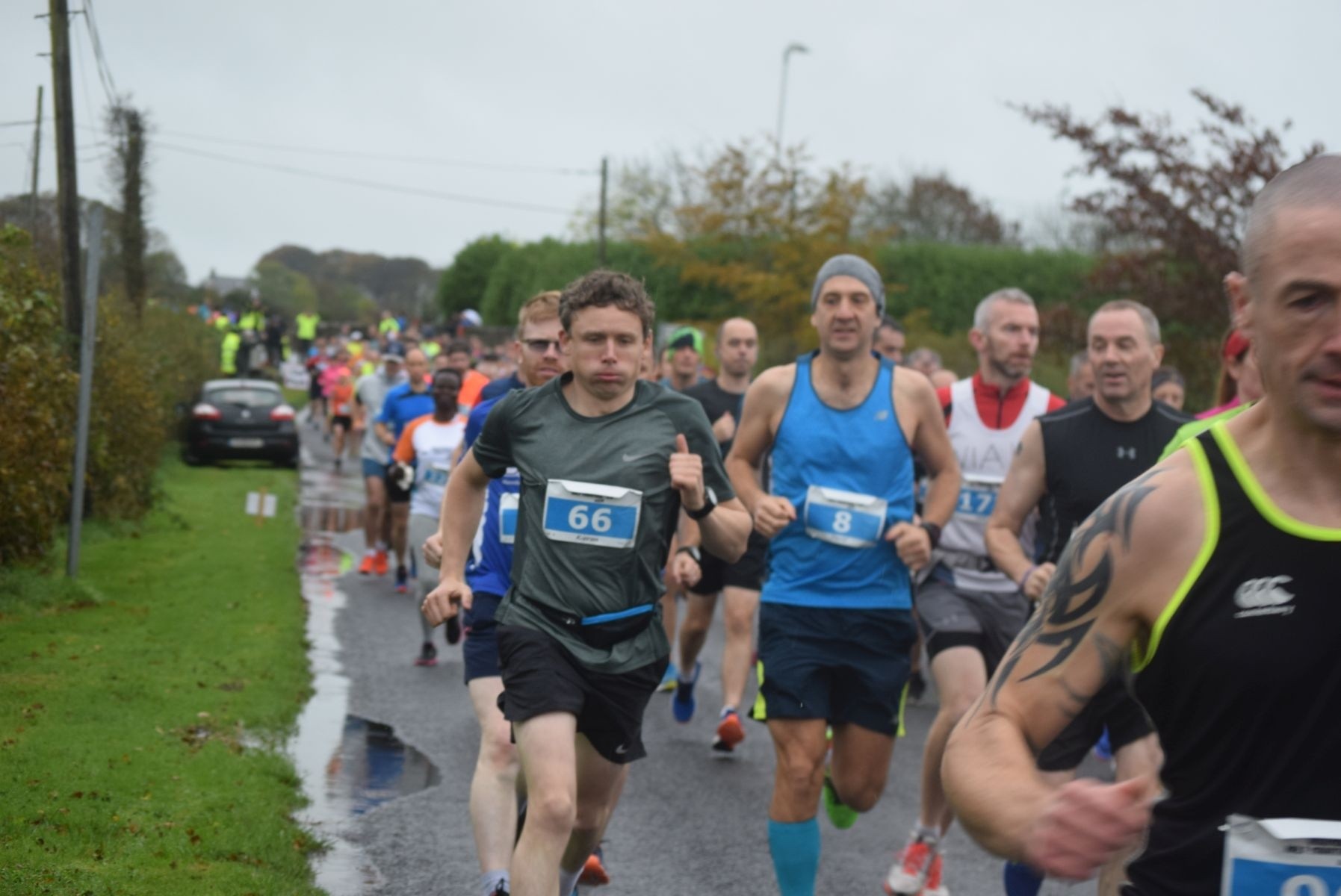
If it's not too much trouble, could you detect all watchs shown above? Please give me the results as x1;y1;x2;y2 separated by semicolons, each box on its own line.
676;545;700;562
921;523;941;549
684;486;718;519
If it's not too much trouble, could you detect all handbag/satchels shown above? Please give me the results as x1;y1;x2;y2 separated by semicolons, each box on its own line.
542;604;659;648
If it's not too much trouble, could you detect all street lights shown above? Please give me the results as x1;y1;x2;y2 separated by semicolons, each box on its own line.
774;42;811;171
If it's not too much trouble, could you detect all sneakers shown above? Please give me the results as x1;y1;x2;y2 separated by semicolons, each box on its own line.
412;641;438;666
444;615;459;644
1003;857;1045;896
821;730;862;827
713;708;745;753
359;556;375;575
577;844;609;886
671;661;701;723
922;853;950;896
374;552;389;575
394;565;408;594
886;825;941;896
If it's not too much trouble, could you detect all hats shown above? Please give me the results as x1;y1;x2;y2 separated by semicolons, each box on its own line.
810;254;886;313
669;325;704;363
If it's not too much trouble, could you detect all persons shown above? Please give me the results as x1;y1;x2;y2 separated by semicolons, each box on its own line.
1195;325;1267;418
421;269;754;896
874;319;957;696
641;316;761;753
884;288;1068;896
1151;366;1184;412
194;295;290;376
985;300;1200;896
293;307;525;666
420;292;608;896
1069;354;1095;400
939;156;1340;896
724;255;962;896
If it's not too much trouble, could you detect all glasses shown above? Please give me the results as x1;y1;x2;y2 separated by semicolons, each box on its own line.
523;339;560;351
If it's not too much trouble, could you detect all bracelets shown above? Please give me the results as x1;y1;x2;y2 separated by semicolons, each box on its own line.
1020;567;1036;590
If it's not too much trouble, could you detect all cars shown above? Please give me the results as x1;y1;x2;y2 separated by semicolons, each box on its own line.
176;380;304;468
